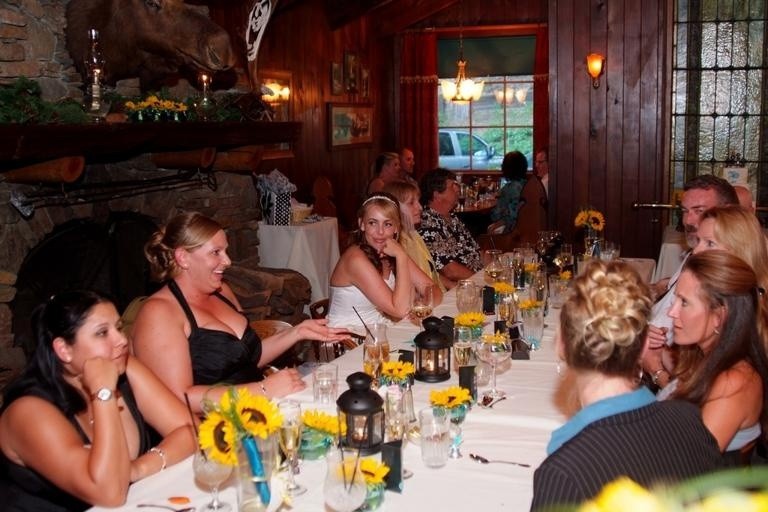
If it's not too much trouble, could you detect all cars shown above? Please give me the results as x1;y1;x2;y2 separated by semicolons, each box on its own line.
417;406;450;469
323;448;367;512
311;362;339;404
455;172;499;207
365;323;391;363
412;230;623;406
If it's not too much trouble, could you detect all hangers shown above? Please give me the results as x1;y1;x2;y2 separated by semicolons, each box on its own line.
469;452;531;468
136;501;195;512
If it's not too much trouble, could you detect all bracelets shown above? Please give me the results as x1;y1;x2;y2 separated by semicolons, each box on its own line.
147;447;167;470
260;383;267;394
651;370;664;386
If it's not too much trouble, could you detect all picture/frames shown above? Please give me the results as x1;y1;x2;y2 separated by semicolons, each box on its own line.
80;252;659;511
255;216;342;300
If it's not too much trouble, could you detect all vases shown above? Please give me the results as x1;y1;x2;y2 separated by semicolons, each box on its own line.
380;361;417;384
575;211;604;242
125;94;187;114
455;264;572;347
431;387;472;408
300;409;350;435
338;457;388;495
194;381;286;503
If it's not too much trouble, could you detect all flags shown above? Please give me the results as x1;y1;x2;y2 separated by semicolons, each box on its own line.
132;109;185;123
435;402;467;423
583;236;599;253
345;482;385;510
293;428;329;457
378;377;410;388
230;436;278;510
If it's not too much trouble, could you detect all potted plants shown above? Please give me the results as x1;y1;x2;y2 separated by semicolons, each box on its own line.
256;70;295;156
325;102;375;151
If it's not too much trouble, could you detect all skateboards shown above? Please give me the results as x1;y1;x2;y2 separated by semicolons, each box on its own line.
66;0;237;89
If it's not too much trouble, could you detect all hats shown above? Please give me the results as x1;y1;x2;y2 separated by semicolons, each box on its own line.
439;129;508;171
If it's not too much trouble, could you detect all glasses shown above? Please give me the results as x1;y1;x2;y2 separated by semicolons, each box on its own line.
414;311;451;383
494;71;529;108
336;366;382;453
586;53;605;89
437;1;486;105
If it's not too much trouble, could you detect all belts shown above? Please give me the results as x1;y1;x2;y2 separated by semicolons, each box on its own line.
192;439;234;512
277;400;308;494
386;387;414;478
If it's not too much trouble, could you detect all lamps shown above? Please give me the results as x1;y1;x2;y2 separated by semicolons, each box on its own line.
247;318;299;385
308;298;366;360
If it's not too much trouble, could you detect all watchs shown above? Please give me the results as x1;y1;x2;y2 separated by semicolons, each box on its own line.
91;388;117;401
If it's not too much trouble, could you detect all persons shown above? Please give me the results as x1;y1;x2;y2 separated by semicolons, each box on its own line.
535;150;548;194
530;262;723;512
651;186;755;294
325;193;442;351
640;204;768;391
382;179;456;293
397;148;416;180
131;211;351;411
658;250;767;452
415;168;491;280
368;152;401;195
4;288;199;511
647;174;739;345
487;151;529;233
